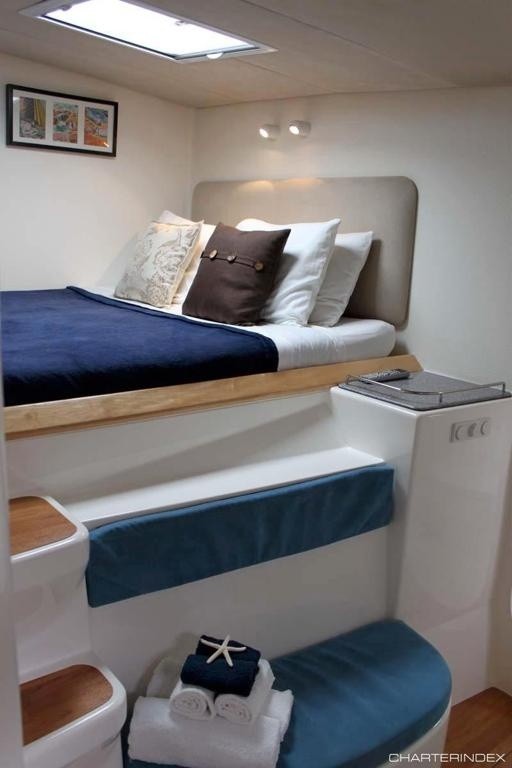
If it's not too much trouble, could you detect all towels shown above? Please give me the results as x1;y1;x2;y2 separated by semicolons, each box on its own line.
124;630;296;767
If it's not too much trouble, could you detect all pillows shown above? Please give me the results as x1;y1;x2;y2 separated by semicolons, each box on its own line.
120;207;376;325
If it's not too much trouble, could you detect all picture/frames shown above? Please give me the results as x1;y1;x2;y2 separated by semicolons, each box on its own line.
2;82;120;155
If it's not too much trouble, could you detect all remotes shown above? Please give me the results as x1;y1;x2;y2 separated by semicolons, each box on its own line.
361;368;410;383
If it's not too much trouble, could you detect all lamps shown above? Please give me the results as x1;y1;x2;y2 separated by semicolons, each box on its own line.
258;125;281;140
288;120;310;136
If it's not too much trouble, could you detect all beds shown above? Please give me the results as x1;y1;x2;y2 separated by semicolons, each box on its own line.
1;176;427;436
